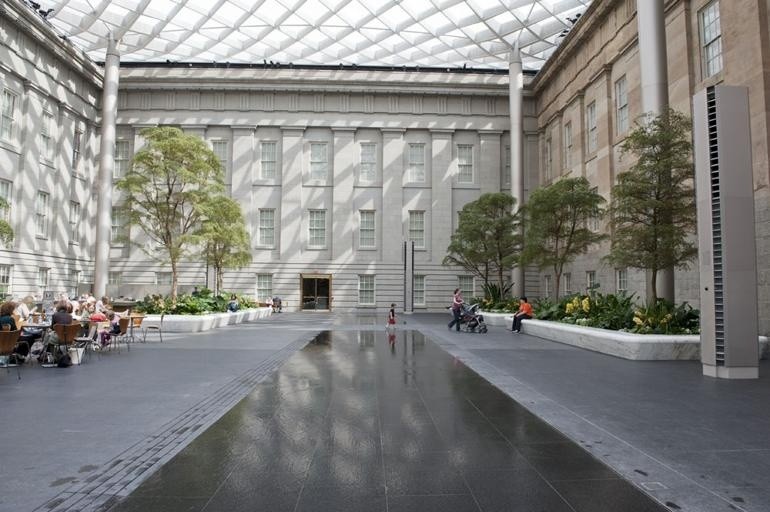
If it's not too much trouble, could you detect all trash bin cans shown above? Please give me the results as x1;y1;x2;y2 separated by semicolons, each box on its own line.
69;348;84;364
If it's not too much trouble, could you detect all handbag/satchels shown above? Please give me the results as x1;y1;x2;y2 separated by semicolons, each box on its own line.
39;347;86;369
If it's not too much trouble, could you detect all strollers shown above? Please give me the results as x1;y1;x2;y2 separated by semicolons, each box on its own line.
460;302;488;334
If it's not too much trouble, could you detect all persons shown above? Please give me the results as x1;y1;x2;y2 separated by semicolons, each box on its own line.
384;303;399;330
224;293;239;313
271;296;283;313
0;290;136;365
508;297;534;333
192;286;199;296
446;288;466;333
265;296;274;307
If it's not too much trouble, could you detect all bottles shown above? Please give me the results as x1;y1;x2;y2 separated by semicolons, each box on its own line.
41;306;50;322
28;314;33;326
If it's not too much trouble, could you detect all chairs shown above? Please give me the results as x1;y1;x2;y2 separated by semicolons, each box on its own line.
0;309;165;379
